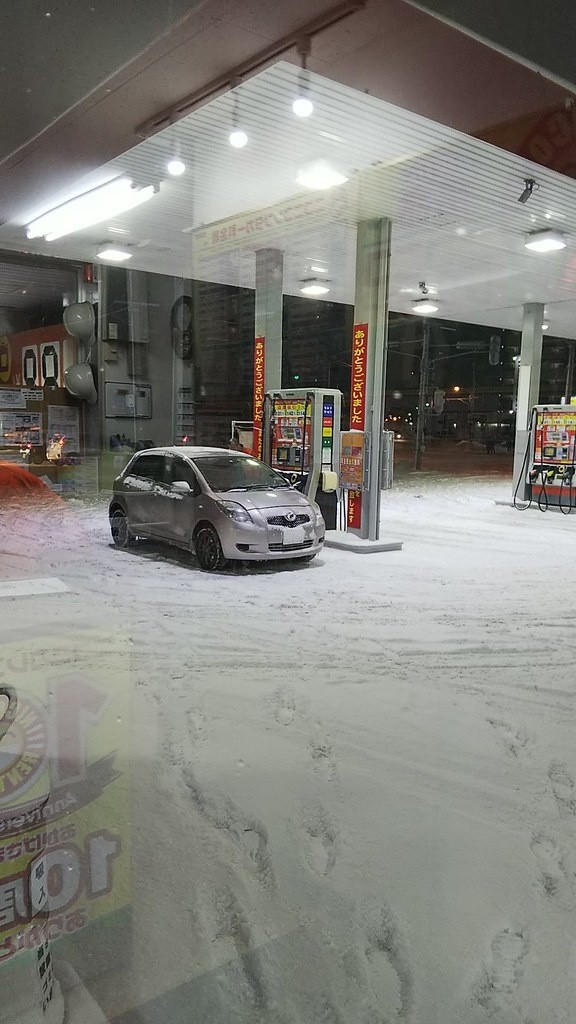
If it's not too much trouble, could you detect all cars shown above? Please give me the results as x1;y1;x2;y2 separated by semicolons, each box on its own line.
107;444;326;571
